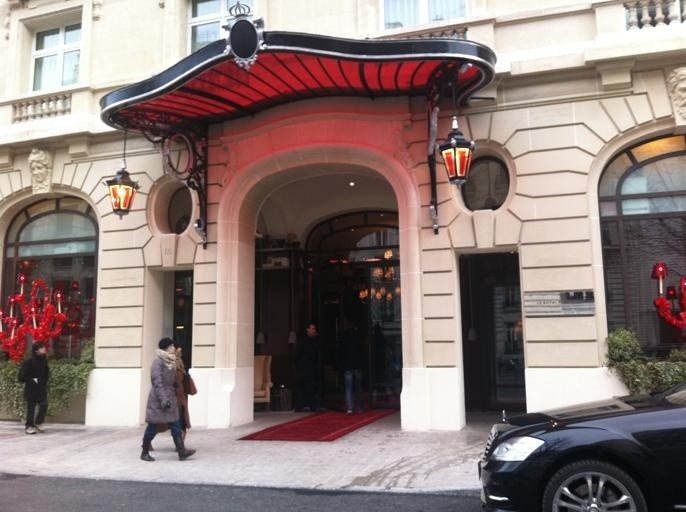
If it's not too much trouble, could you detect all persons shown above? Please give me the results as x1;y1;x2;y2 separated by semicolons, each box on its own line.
27;149;52;185
288;322;320;412
336;316;367;415
140;337;197;462
666;66;686;119
173;344;197;452
20;341;50;433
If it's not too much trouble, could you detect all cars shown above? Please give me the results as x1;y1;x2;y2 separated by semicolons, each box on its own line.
477;377;686;511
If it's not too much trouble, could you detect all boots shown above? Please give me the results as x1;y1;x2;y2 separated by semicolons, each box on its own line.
142;437;155;461
172;431;196;460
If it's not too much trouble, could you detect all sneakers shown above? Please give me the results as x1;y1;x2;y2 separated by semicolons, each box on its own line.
25;424;44;434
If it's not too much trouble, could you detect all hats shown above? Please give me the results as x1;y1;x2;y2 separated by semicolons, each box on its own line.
159;337;182;350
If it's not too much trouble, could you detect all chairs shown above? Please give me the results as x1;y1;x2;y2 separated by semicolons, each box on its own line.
254;355;273;412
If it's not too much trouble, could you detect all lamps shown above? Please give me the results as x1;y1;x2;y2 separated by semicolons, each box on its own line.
255;331;266;344
437;79;476;189
68;282;96;329
666;286;676;311
651;261;668;297
288;331;297;343
0;272;68;362
359;287;400;304
102;129;142;220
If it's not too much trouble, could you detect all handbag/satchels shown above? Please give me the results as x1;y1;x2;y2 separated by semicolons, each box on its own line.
184;368;197;395
